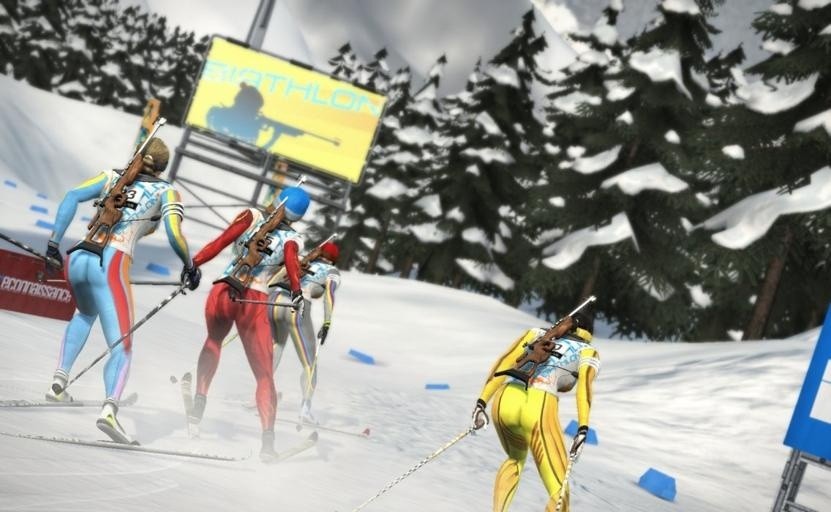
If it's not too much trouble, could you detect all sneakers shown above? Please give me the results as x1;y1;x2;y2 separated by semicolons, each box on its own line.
96;398;136;445
46;369;72;402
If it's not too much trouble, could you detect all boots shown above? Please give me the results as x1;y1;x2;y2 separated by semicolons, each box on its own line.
188;394;206;424
260;431;278;461
300;399;317;425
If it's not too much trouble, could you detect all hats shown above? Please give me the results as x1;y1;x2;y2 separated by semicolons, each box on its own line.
568;313;593;343
320;242;338;264
272;186;310;221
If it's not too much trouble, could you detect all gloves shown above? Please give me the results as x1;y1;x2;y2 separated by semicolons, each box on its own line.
471;399;489;430
570;425;588;460
181;264;200;290
44;244;64;277
179;267;188;284
291;290;305;320
317;324;328;344
